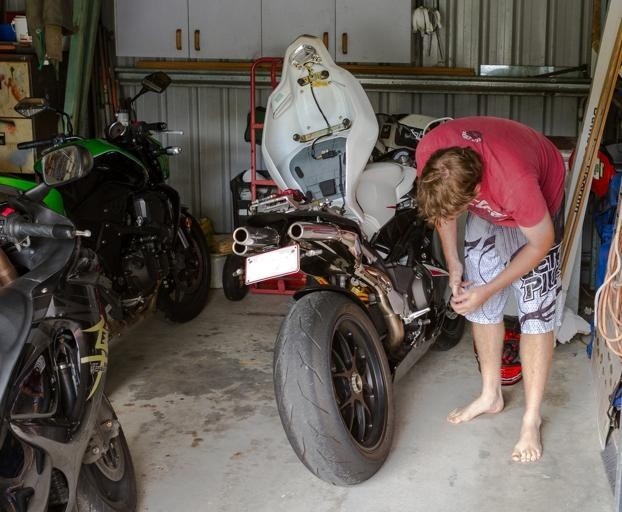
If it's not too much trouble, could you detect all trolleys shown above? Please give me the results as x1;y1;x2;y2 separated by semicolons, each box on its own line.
222;56;316;300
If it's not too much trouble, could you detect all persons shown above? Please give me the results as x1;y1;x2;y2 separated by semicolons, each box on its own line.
415;117;566;462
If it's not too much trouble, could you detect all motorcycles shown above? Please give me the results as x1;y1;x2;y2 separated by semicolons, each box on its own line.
232;34;465;487
0;72;212;324
1;143;136;508
